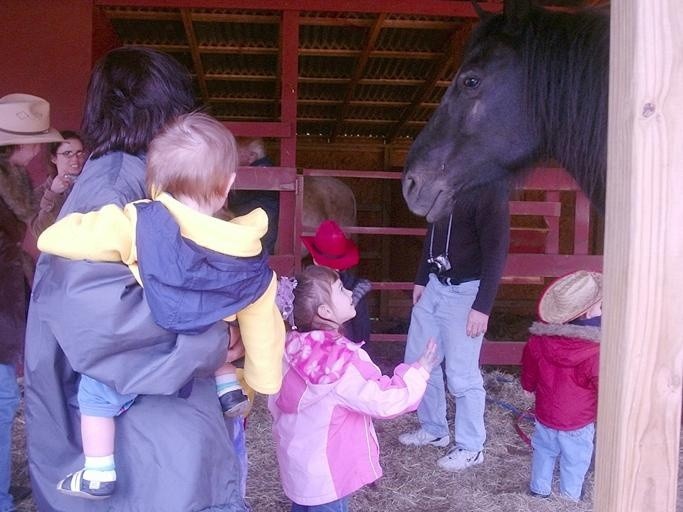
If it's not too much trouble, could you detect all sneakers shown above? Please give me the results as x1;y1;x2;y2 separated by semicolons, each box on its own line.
437;448;484;471
58;469;114;501
398;429;450;448
219;390;251;417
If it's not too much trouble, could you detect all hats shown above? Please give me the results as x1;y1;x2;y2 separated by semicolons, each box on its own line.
300;219;360;271
536;269;603;324
0;92;70;148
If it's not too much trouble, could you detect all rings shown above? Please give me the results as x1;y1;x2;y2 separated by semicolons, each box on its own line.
64;174;68;178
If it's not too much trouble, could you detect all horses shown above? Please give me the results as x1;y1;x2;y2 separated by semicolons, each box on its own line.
402;0;611;224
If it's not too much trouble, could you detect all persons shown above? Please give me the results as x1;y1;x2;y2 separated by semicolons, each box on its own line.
518;271;603;505
0;91;65;512
268;265;439;512
23;47;249;512
37;112;286;500
31;126;88;235
398;178;509;475
223;136;279;257
300;221;370;351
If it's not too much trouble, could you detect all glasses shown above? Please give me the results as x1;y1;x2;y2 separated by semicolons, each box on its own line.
56;151;87;160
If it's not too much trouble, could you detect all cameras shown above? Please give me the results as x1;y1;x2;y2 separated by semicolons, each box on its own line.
427;254;451;275
64;174;78;192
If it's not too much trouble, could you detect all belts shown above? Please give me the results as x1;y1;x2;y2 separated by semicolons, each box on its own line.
437;275;482;286
